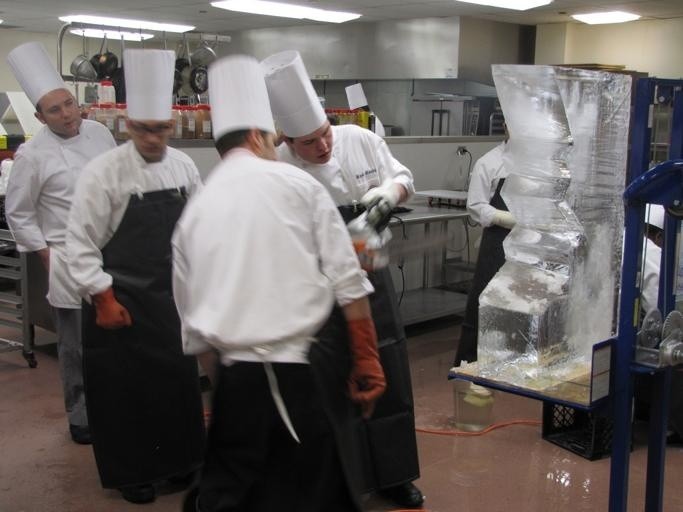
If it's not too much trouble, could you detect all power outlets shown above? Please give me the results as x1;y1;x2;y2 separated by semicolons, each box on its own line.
457;146;466;156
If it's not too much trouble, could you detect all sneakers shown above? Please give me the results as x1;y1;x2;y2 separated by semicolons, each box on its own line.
68;419;94;445
120;480;156;505
377;478;424;508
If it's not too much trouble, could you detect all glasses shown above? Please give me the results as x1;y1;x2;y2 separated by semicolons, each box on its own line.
130;123;149;135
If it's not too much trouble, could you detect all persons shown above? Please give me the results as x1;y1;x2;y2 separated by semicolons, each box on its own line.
1;89;120;447
441;118;522;383
624;220;670;321
166;125;388;509
60;116;204;506
270;119;426;512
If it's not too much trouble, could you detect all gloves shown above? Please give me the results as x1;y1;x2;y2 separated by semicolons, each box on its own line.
491;206;518;232
91;286;134;333
343;314;389;421
360;178;401;228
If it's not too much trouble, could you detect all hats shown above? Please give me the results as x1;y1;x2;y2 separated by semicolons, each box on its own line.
6;38;68;109
121;48;176;122
260;48;329;145
345;81;369;111
205;53;279;147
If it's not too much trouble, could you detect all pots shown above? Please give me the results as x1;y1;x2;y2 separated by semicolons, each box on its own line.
88;34;118;83
173;40;216;95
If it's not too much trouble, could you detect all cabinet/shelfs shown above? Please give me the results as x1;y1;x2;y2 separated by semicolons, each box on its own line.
386;190;481;328
1;228;40;368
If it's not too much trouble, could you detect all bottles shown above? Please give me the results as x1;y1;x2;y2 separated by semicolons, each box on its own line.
78;80;214;141
